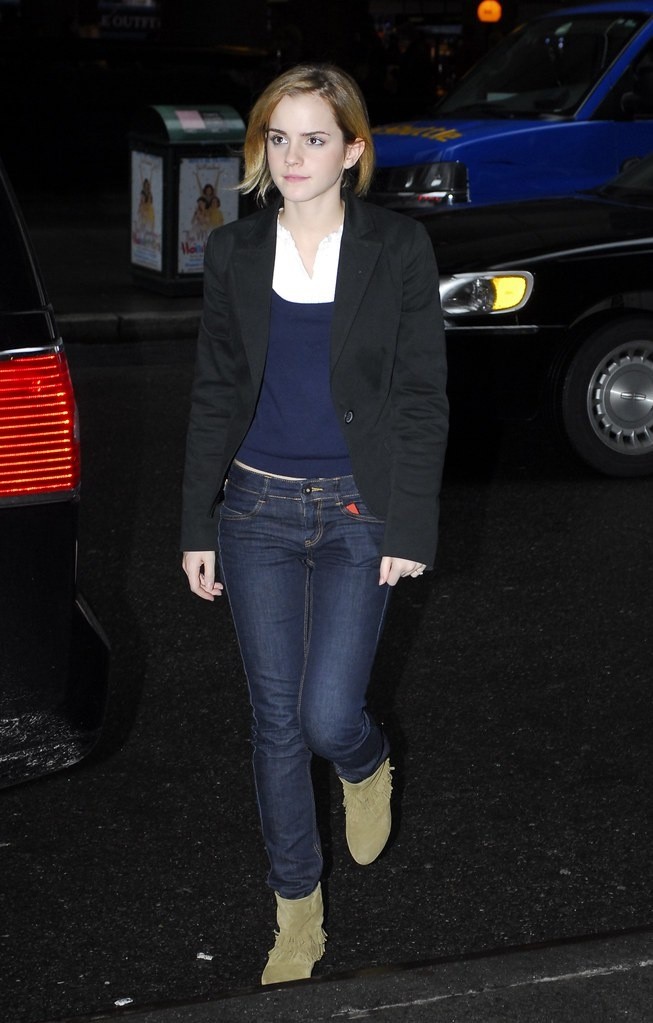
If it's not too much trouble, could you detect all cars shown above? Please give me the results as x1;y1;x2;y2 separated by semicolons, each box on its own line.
409;154;653;480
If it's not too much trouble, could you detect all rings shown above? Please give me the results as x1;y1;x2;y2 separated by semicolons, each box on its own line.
416;570;423;575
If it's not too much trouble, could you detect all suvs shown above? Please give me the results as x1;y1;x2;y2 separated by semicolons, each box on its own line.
362;4;653;209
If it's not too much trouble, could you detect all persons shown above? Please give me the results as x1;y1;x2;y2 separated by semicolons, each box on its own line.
393;34;439;99
136;164;155;233
180;67;448;987
192;168;224;244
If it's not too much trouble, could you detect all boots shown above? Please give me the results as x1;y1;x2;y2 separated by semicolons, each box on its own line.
338;759;393;866
261;882;327;986
